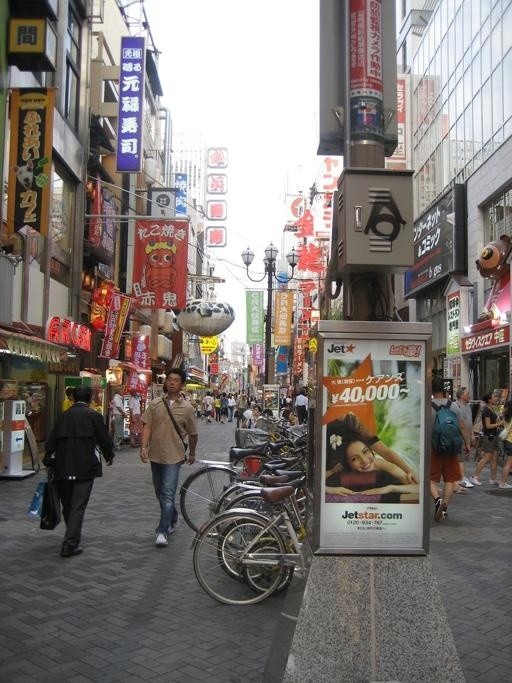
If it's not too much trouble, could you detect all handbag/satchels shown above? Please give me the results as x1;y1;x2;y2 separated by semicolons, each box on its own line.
54;463;85;481
41;482;60;529
28;480;44;518
500;429;510;441
480;435;498;452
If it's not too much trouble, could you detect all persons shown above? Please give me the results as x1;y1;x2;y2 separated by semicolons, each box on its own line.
202;390;309;429
140;369;198;547
129;388;143;448
112;388;127;451
431;368;511;522
45;385;116;558
62;388;74;411
323;413;422;500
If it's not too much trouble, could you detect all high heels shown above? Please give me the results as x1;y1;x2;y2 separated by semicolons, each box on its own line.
60;546;82;557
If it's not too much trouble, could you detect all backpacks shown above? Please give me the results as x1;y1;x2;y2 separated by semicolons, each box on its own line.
432;407;464;457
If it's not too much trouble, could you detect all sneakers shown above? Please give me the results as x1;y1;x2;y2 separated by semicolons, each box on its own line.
435;499;447;521
442;503;449;521
156;530;168;547
453;475;508;492
167;514;180;532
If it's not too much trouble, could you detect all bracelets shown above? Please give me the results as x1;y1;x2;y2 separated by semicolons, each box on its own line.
141;448;147;449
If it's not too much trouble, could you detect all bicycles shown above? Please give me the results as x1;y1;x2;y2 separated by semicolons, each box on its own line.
176;411;312;605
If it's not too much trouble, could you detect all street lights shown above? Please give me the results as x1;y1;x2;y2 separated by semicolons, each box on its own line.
242;241;299;382
142;152;159;217
